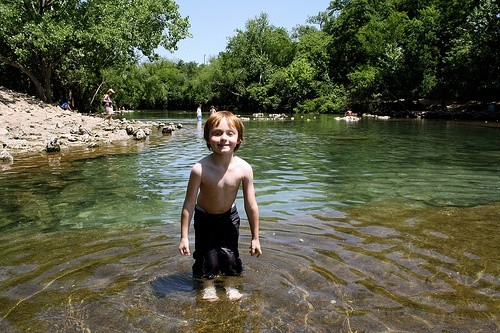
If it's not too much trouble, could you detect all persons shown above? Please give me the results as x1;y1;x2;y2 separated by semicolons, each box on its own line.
179;110;262;277
102;89;115;124
61;101;71;109
196;104;202;120
210;106;216;115
